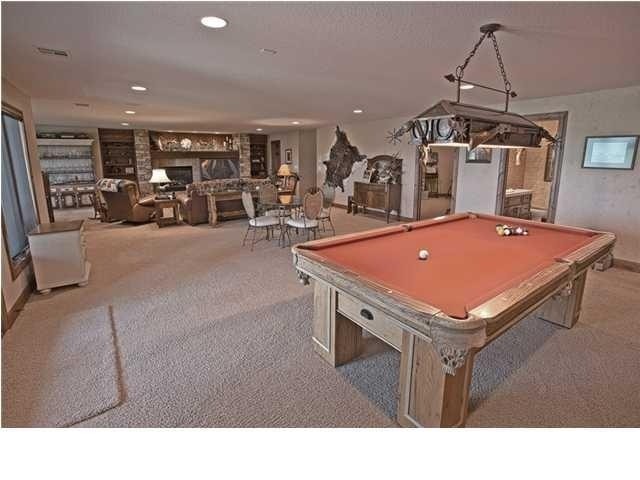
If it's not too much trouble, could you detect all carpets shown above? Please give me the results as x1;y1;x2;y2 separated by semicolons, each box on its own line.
1;305;121;428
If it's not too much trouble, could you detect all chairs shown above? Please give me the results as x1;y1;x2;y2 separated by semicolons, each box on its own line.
283;192;322;248
302;182;336;238
243;191;284;252
260;184;278;237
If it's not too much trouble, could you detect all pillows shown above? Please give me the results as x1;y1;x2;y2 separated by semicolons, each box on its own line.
138;197;153;206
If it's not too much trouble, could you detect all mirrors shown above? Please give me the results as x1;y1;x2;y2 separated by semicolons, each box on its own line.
367;155;403;183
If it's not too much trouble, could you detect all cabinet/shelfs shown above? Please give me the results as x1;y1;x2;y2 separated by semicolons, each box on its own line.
35;138;96;184
49;185;59;209
75;184;96;208
248;135;269;180
502;189;535;219
59;184;76;209
426;152;439;197
25;220;91;296
97;129;137;183
353;180;402;224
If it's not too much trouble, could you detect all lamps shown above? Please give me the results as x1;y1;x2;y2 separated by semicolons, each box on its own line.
402;23;557;148
278;164;292;192
147;167;174;200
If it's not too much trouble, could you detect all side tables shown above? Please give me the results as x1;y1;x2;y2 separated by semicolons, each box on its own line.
154;199;180;229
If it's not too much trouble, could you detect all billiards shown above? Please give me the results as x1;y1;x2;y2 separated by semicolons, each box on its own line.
418;249;429;260
496;224;529;235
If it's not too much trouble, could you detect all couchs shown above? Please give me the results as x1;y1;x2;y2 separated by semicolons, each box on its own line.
97;177;163;223
178;178;272;225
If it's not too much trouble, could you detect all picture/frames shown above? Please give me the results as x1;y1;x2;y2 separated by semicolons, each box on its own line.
465;147;492;164
285;148;292;164
544;145;556;182
582;134;640;170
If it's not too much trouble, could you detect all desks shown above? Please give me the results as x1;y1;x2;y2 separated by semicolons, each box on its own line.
207;190;259;227
258;195;305;239
